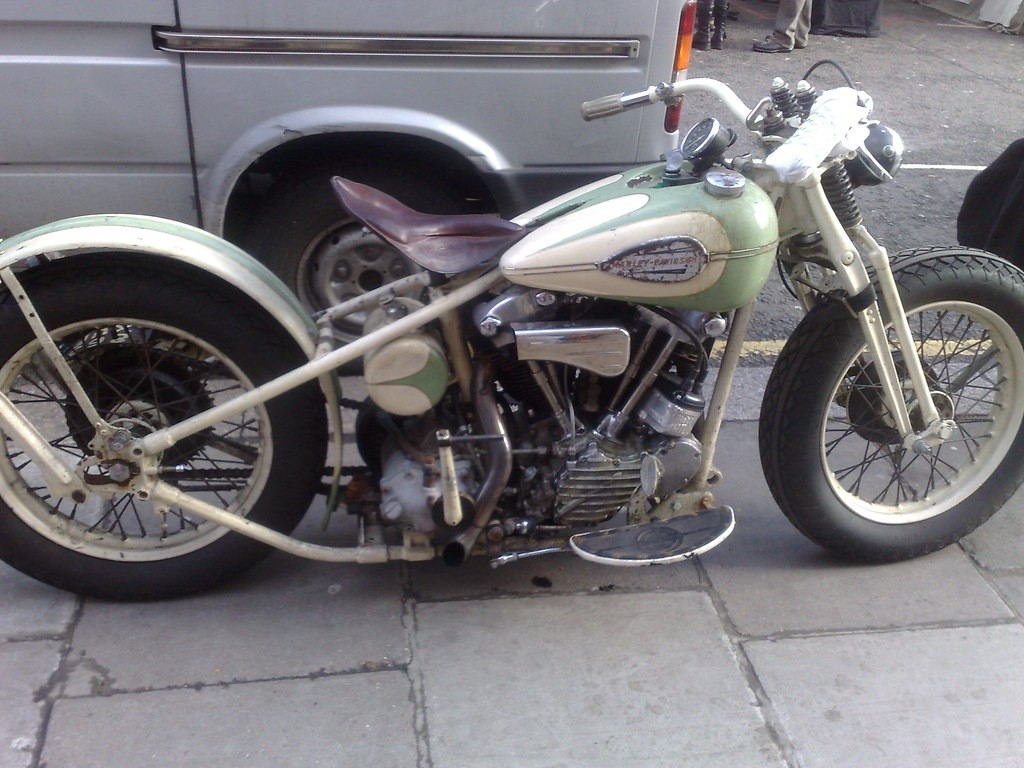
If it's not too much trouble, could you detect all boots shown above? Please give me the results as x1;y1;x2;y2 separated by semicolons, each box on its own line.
710;0;728;50
692;0;714;50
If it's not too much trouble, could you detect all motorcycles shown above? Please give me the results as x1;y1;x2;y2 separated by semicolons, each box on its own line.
0;58;1024;602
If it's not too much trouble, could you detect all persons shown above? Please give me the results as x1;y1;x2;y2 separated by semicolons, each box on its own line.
691;0;730;50
752;0;813;52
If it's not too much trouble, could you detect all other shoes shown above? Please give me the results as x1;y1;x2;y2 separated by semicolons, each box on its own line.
753;38;791;52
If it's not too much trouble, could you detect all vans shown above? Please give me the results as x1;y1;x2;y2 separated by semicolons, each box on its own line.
0;1;701;374
